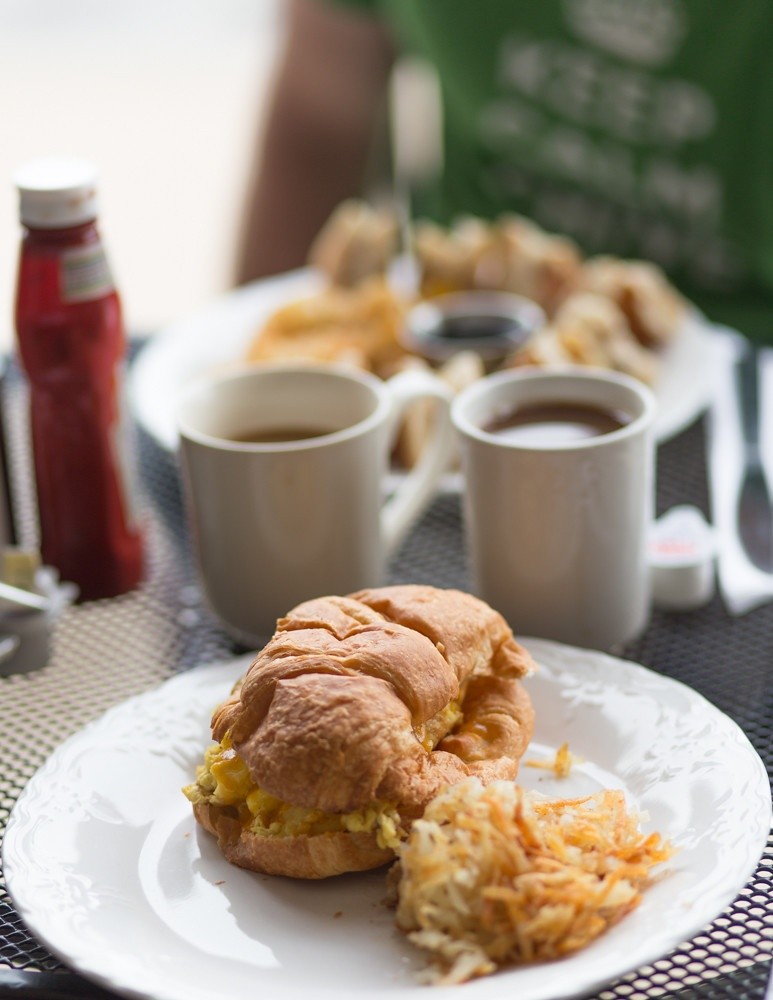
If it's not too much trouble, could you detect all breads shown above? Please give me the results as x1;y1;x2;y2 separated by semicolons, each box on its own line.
246;197;691;470
182;583;536;878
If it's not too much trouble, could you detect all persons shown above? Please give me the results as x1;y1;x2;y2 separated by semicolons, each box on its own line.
230;0;772;356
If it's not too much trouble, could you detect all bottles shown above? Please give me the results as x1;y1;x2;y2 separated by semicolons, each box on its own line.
6;170;150;604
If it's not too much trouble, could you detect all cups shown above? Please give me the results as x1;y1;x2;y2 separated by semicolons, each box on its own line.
393;287;543;376
171;364;451;648
449;364;657;654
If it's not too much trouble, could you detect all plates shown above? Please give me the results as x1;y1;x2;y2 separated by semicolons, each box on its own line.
124;261;732;496
1;634;773;1000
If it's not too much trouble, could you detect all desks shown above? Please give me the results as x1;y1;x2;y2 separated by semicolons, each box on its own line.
0;338;773;1000
